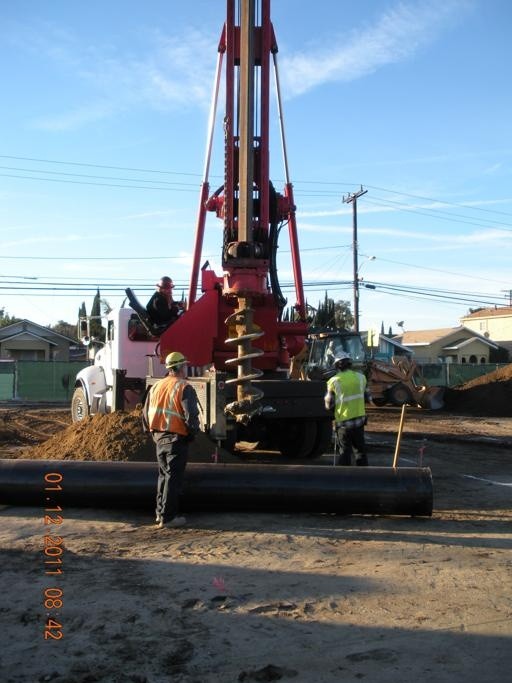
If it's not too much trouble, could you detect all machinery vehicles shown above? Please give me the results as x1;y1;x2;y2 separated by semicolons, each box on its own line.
298;326;452;412
68;0;350;461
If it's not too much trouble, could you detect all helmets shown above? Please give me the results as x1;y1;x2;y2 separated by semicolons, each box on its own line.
333;352;350;366
156;277;175;289
166;351;190;368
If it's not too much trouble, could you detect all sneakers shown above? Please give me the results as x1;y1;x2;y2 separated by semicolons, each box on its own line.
156;516;186;528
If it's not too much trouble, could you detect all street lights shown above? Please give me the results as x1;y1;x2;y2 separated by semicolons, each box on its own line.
351;253;378;331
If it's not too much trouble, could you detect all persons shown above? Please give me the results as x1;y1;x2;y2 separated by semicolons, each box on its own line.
325;351;368;466
146;276;181;336
142;352;200;529
